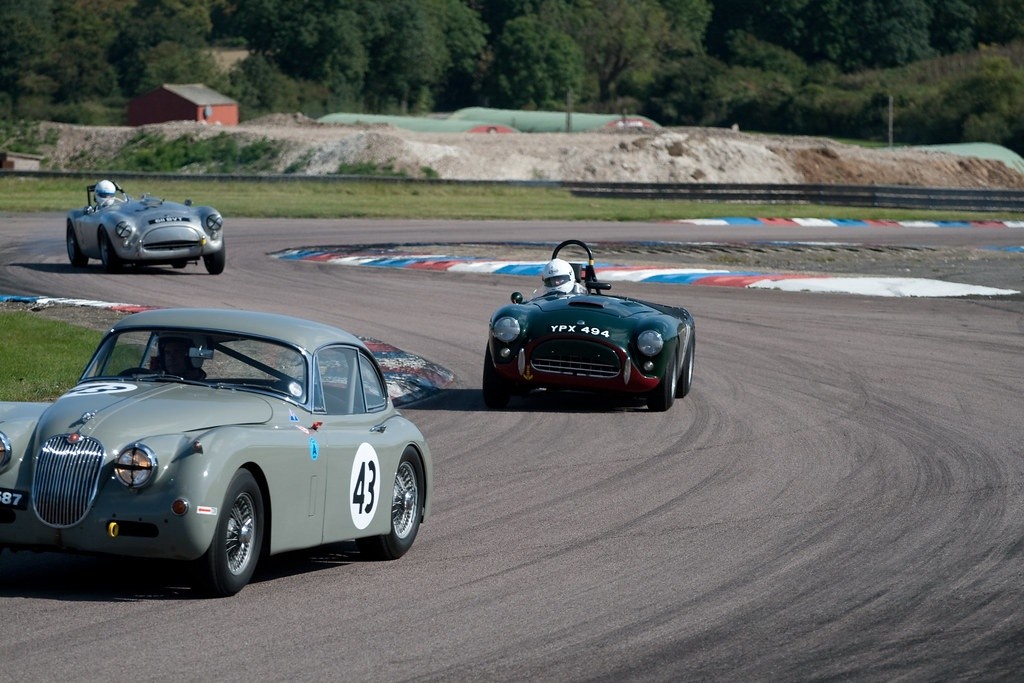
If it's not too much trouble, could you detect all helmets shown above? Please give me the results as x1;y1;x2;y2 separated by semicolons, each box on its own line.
94;180;116;205
541;258;576;294
154;331;207;368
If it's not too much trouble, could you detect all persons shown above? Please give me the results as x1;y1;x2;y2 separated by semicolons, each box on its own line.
94;180;115;206
150;331;207;381
542;259;588;298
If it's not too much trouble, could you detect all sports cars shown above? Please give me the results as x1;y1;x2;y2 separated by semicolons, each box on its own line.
66;181;226;275
1;308;431;597
483;238;696;412
